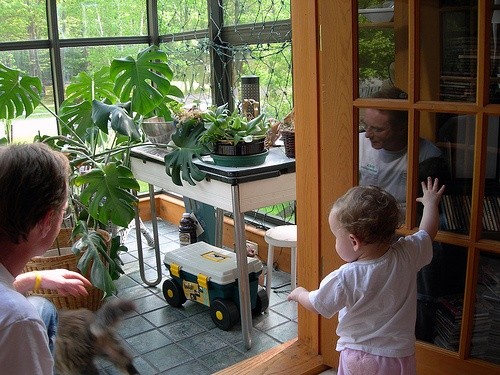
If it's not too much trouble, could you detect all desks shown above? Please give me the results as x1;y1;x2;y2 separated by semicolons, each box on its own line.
129;143;297;351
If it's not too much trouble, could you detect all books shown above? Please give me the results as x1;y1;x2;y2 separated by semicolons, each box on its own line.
431;36;500;364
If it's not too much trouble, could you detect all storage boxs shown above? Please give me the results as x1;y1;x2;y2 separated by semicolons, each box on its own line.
164;240;264;306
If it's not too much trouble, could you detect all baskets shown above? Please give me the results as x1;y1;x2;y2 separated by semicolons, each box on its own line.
22;228;111;315
281;129;296;158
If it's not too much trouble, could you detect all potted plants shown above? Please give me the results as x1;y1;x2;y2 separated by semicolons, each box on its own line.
142;98;218;147
280;122;296;158
0;44;185;311
202;102;270;155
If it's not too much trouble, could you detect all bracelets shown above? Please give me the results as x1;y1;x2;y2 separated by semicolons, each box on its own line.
33;270;42;291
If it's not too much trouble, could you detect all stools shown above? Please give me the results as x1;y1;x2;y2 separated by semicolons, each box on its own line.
265;225;297;315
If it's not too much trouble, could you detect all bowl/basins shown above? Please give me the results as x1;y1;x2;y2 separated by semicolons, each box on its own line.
140;115;175;149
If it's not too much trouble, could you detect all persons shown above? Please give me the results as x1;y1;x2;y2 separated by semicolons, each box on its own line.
287;177;446;375
0;142;93;375
357;88;457;344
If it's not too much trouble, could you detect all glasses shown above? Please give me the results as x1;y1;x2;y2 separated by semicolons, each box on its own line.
56;205;72;219
363;124;391;134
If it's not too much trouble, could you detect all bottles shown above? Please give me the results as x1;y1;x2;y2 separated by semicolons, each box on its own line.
178;214;196;247
